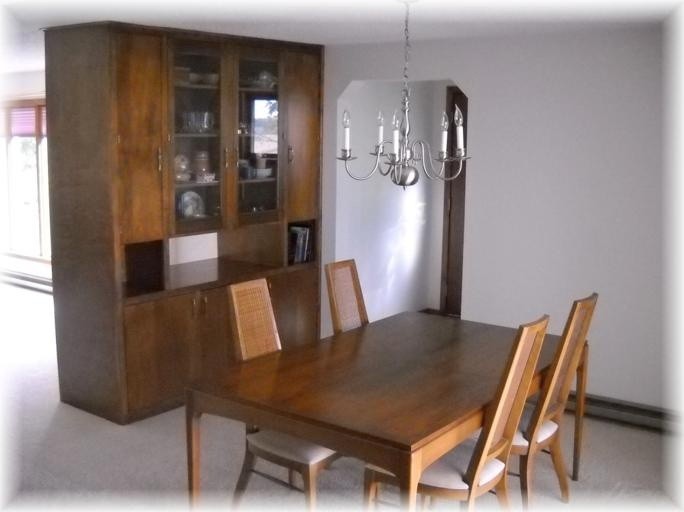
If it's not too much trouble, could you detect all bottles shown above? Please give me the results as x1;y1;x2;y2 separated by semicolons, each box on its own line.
190;149;210;174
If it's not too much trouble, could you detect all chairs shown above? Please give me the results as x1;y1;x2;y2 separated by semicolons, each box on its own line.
225;257;369;509
360;310;552;506
468;291;598;505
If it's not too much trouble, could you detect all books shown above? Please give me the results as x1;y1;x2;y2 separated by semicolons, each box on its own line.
287;225;316;263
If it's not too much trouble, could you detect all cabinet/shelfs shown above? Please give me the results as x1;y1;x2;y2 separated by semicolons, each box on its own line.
39;22;326;427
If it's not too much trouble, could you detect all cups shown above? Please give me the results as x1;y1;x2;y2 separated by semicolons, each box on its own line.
242;166;258;180
256;156;266;169
188;71;219;85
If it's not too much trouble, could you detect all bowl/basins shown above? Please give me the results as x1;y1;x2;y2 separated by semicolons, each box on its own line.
191;171;217;184
180;108;215;134
255;168;273;179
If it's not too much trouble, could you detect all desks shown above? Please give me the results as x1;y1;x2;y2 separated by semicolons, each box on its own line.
181;308;590;507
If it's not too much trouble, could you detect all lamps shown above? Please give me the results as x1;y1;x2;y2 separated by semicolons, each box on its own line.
334;1;469;191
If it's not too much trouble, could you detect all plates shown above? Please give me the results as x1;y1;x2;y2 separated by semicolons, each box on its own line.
179;191;207;221
